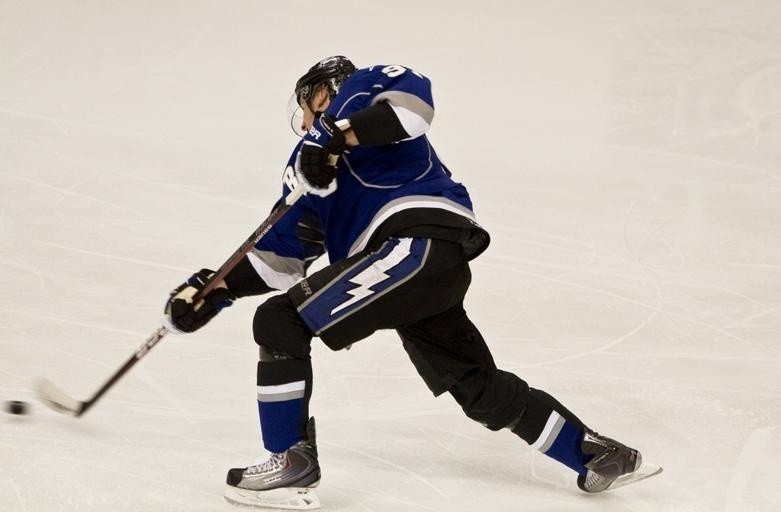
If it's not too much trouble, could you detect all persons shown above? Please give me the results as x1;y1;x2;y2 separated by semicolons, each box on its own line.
162;50;642;495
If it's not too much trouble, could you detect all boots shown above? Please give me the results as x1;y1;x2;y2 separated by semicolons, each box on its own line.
576;428;643;493
226;416;322;492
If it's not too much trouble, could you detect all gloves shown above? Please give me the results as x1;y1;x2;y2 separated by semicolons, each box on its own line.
295;113;346;197
161;267;238;335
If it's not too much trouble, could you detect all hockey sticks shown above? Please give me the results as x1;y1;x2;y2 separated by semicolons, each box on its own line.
32;152;340;417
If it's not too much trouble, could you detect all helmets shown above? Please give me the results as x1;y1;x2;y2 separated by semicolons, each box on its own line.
294;55;360;108
